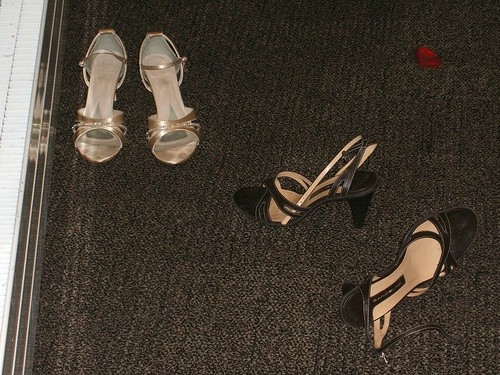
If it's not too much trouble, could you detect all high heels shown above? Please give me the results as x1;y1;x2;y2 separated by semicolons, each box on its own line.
233;135;379;230
139;31;200;165
341;206;478;349
71;28;128;162
